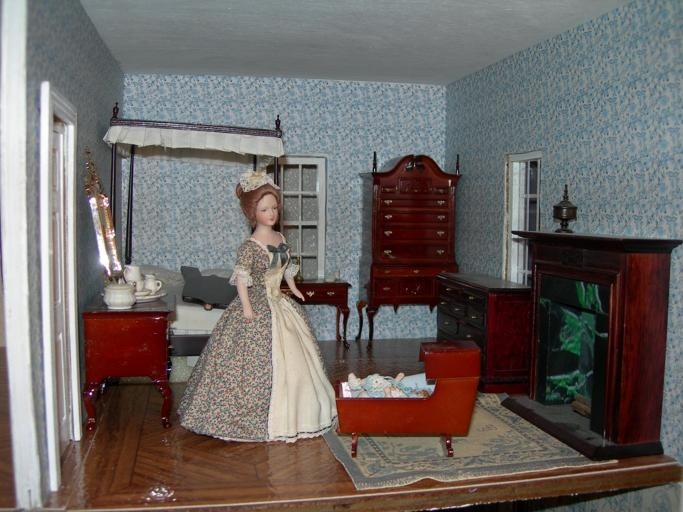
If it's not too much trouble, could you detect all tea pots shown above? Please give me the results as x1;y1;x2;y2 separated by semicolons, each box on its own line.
102;280;137;310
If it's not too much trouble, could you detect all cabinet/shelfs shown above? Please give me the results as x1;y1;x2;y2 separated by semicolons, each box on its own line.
81;289;175;434
500;231;682;463
356;150;464;340
434;272;532;394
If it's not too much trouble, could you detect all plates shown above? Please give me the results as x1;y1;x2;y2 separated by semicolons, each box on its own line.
132;290;151;296
99;289;166;303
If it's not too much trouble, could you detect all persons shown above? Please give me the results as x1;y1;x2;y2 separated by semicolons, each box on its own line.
177;171;336;442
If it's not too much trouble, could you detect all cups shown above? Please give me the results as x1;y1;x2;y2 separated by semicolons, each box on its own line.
143;274;162;295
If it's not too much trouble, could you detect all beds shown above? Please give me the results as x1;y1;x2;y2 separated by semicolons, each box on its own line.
103;101;287;348
336;340;481;459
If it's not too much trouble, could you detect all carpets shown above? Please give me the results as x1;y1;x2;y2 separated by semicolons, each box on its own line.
321;387;619;491
